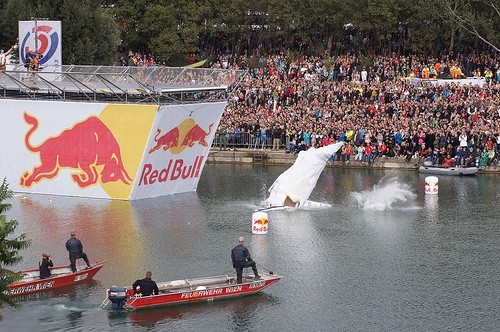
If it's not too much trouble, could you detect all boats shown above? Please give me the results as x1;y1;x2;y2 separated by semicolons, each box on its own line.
99;273;283;309
417;162;478;175
2;265;103;298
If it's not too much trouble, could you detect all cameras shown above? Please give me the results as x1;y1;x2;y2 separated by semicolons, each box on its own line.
47;254;50;260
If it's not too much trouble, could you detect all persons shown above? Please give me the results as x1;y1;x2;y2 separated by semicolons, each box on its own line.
39;254;53;279
132;271;158;297
119;45;500;169
0;46;14;70
66;231;92;273
231;236;261;284
24;50;39;76
